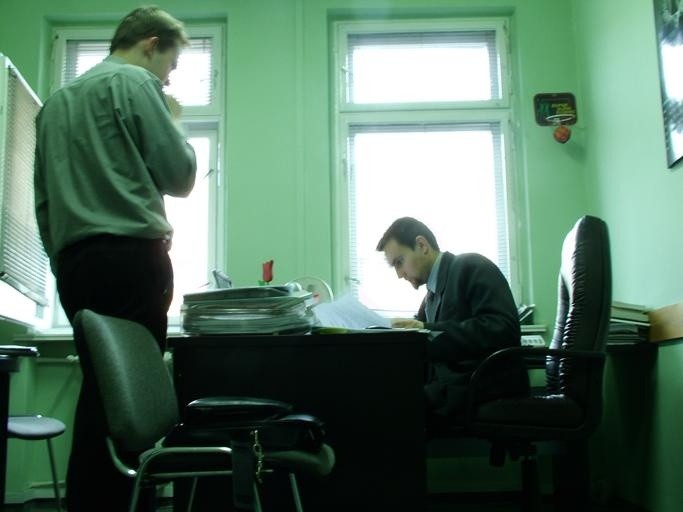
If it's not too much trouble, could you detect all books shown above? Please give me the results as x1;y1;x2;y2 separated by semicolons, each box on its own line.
0;342;39;358
182;283;315;337
603;302;652;347
514;302;546;336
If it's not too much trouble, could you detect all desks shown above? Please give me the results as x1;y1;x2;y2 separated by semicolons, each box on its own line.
168;325;431;510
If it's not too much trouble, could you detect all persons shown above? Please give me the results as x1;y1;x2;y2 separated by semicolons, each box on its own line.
30;5;199;512
374;215;523;511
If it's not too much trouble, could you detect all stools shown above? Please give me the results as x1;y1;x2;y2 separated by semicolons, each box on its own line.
8;415;66;512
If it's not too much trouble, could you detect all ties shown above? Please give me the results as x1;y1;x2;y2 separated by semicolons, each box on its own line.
425;292;433;322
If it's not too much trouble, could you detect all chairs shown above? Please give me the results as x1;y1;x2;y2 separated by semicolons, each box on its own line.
411;216;613;511
73;307;324;511
